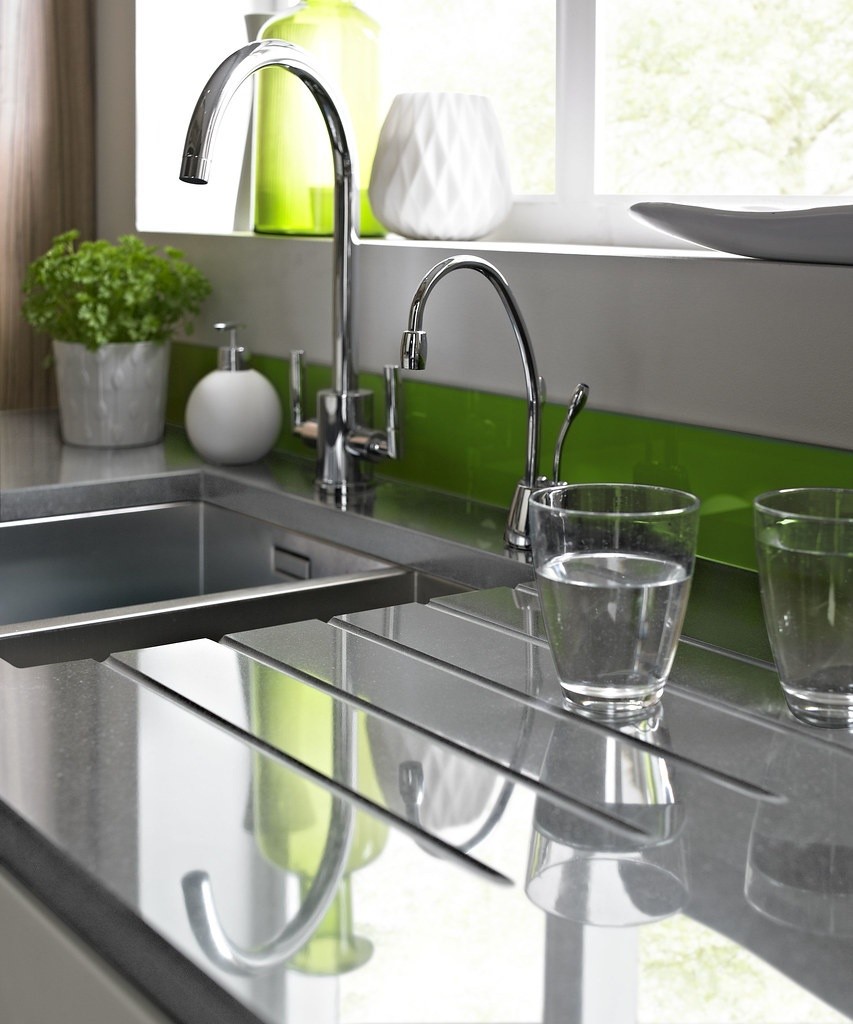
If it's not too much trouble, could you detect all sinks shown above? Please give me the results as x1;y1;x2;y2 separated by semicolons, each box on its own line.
0;497;418;671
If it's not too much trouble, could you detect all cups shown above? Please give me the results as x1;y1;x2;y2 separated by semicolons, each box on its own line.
369;93;510;241
526;483;699;717
754;487;853;729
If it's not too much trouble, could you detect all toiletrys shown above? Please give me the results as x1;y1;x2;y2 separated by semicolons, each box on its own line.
183;319;283;465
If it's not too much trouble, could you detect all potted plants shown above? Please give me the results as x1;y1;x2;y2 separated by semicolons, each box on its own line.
22;229;212;446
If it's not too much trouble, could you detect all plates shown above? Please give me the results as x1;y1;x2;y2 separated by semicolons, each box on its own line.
630;202;853;265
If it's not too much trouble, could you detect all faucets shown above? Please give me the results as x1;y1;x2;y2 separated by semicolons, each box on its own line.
180;607;354;978
176;38;376;500
400;586;541;862
396;252;542;550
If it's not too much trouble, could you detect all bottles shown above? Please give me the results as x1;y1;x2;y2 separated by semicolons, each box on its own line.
254;0;381;235
